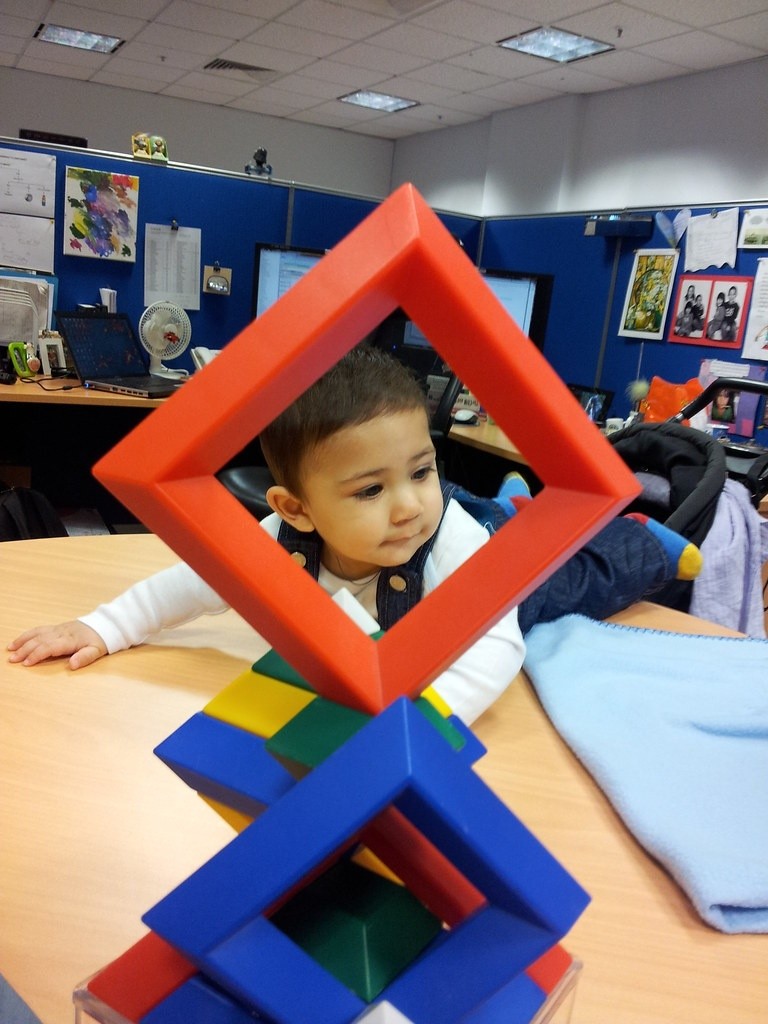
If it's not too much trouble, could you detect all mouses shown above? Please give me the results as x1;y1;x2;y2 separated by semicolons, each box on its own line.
455;409;479;424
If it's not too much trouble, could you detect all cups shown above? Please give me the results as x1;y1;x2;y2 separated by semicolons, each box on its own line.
605;417;626;435
703;423;730;440
77;304;97;314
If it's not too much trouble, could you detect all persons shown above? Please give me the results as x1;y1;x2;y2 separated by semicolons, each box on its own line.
14;348;26;371
712;389;735;423
584;394;605;421
8;346;703;730
47;348;56;368
706;286;739;341
676;285;705;337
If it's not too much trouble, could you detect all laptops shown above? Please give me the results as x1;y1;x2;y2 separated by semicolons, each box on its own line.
52;311;187;398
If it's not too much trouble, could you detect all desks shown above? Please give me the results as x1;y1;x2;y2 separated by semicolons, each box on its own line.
1;374;528;467
3;532;768;1024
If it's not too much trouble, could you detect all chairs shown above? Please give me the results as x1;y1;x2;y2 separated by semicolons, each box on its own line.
216;370;465;523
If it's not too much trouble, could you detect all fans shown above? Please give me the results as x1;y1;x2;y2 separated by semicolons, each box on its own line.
138;300;191;379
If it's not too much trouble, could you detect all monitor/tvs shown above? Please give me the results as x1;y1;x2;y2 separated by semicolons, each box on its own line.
254;242;554;381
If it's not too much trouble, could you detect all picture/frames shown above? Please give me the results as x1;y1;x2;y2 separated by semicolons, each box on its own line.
617;248;680;340
39;337;68;376
566;382;615;430
130;129;169;161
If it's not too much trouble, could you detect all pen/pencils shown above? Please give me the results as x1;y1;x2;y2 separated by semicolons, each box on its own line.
636;402;641;412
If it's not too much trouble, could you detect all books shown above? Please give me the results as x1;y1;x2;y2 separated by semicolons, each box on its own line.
99;288;117;313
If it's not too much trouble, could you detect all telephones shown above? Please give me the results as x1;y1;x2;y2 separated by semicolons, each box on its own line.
189;347;221;369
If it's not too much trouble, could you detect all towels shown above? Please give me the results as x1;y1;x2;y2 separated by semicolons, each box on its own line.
521;613;768;934
